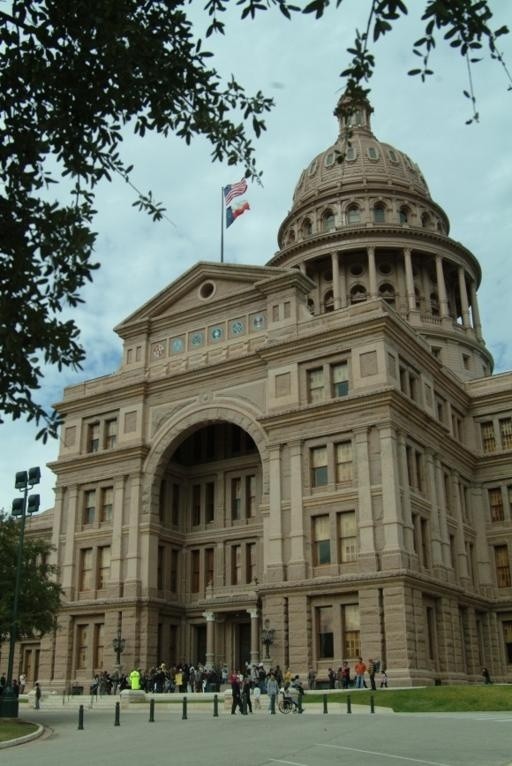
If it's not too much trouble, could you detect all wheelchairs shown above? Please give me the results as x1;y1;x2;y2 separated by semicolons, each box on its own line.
279;692;296;714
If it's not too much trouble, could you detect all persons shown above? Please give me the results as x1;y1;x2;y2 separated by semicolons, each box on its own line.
95;660;228;696
19;671;27;694
34;682;42;710
481;667;493;685
230;660;316;715
12;679;19;698
1;672;7;691
327;656;389;689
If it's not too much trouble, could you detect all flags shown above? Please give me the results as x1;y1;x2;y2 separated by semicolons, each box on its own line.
225;198;251;229
222;177;248;207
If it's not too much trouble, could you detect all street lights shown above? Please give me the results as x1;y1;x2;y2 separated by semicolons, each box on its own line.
261;619;275;658
113;629;125;664
0;467;40;717
